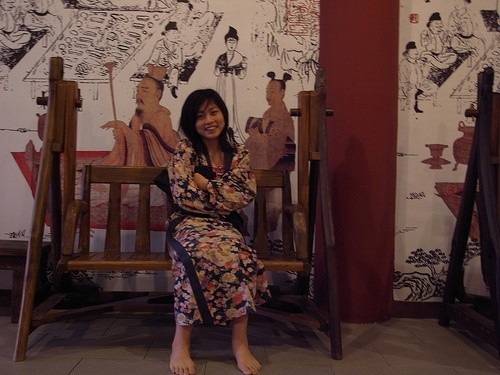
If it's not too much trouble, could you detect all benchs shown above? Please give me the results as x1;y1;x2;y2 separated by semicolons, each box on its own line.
61;165;308;271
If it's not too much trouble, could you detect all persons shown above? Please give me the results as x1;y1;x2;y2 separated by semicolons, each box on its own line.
167;89;272;375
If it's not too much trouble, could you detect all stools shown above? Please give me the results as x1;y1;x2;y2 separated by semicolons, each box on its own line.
0;240;50;323
